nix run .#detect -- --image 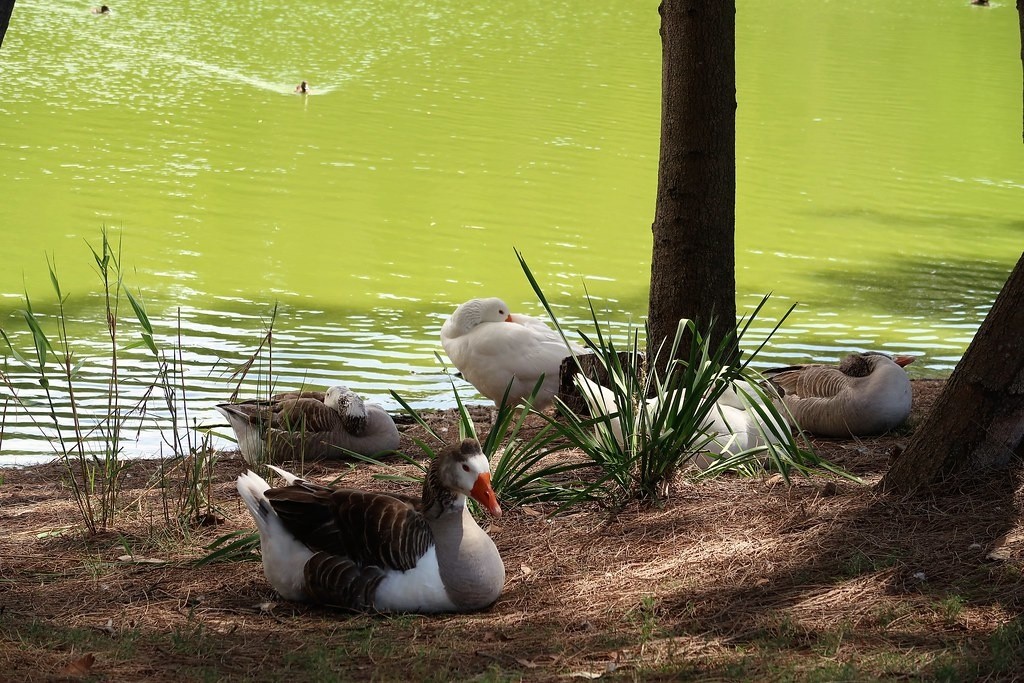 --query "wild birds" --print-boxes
[294,81,309,95]
[235,437,506,615]
[971,0,990,6]
[214,385,400,466]
[757,351,916,438]
[91,6,111,14]
[440,297,610,410]
[571,364,792,474]
